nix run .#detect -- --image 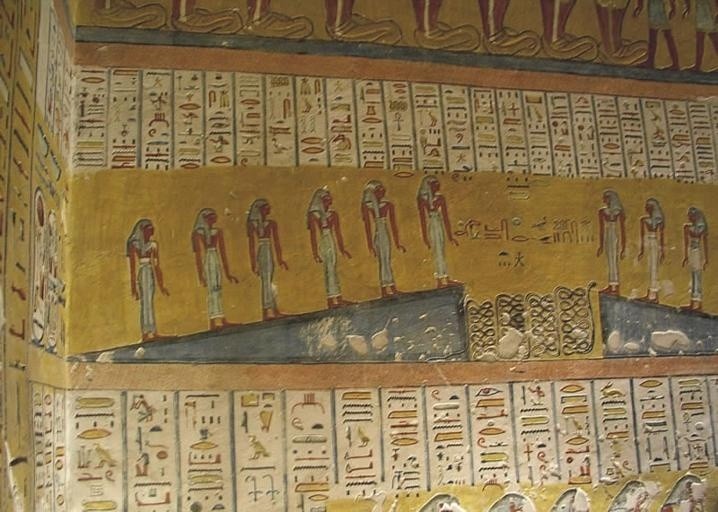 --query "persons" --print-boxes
[633,0,679,71]
[127,218,170,341]
[361,180,406,297]
[417,175,461,288]
[191,209,239,330]
[247,198,288,321]
[307,188,353,309]
[682,0,718,73]
[597,190,625,297]
[637,197,665,305]
[680,206,708,310]
